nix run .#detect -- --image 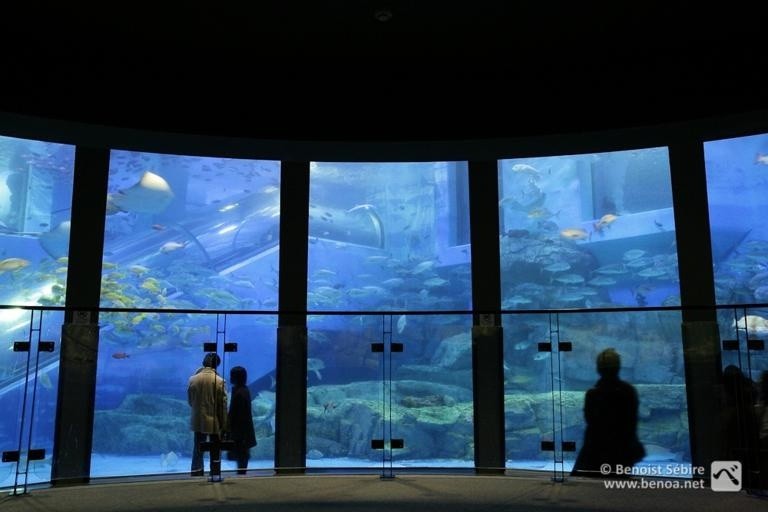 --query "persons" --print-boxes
[220,365,257,475]
[568,347,647,479]
[187,353,230,476]
